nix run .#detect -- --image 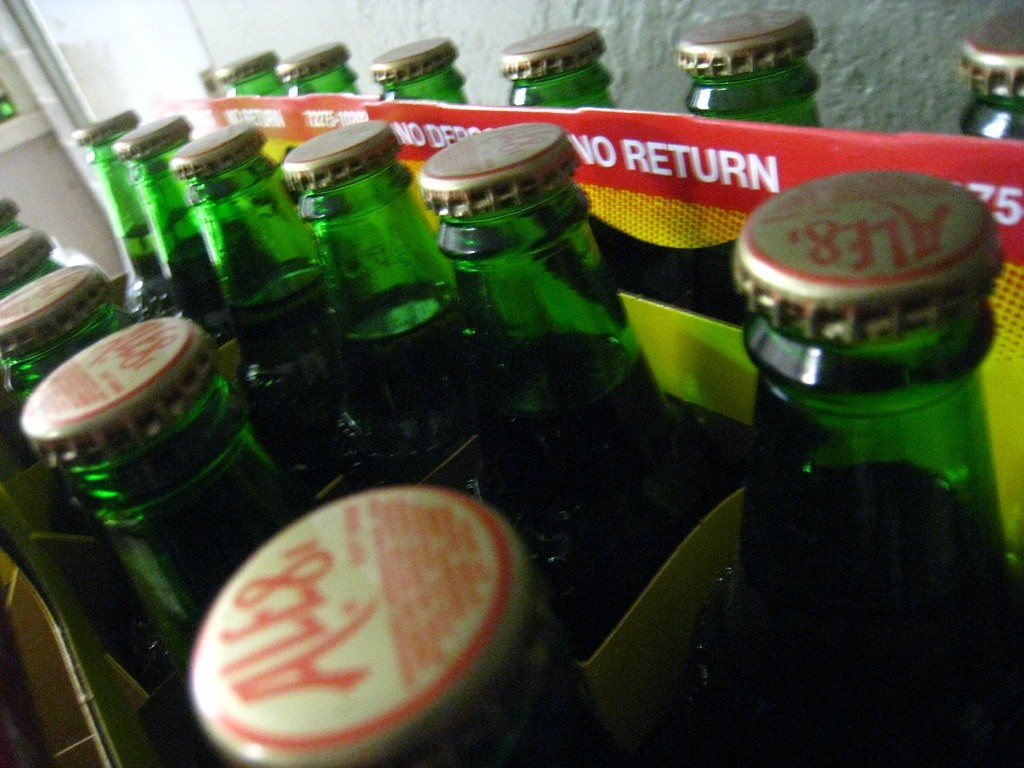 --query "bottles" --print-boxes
[0,10,1024,768]
